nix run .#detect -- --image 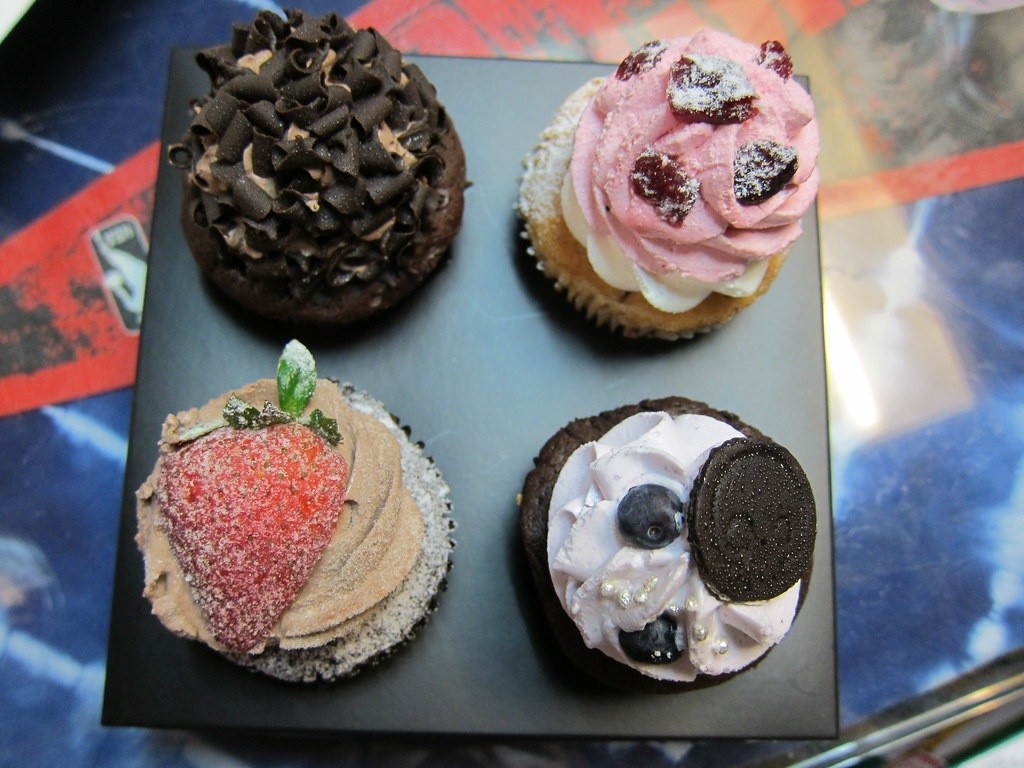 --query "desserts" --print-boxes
[179,7,468,326]
[133,339,455,683]
[519,397,818,692]
[517,26,819,343]
